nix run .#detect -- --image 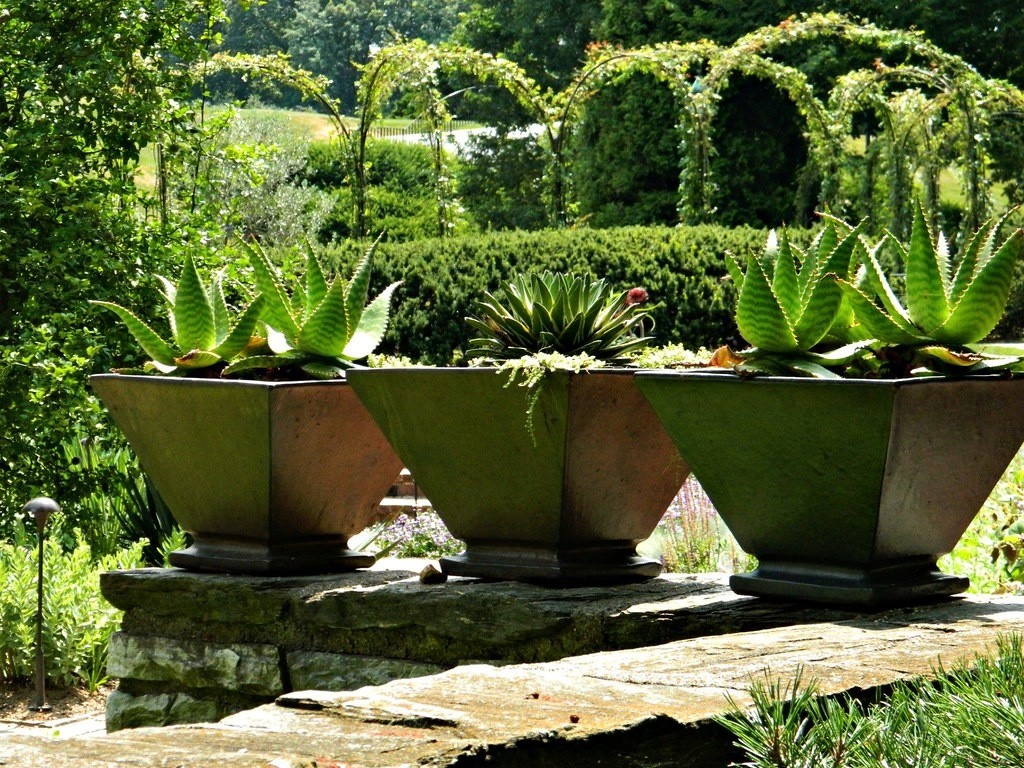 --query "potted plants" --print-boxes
[344,270,693,588]
[87,231,406,577]
[633,196,1023,606]
